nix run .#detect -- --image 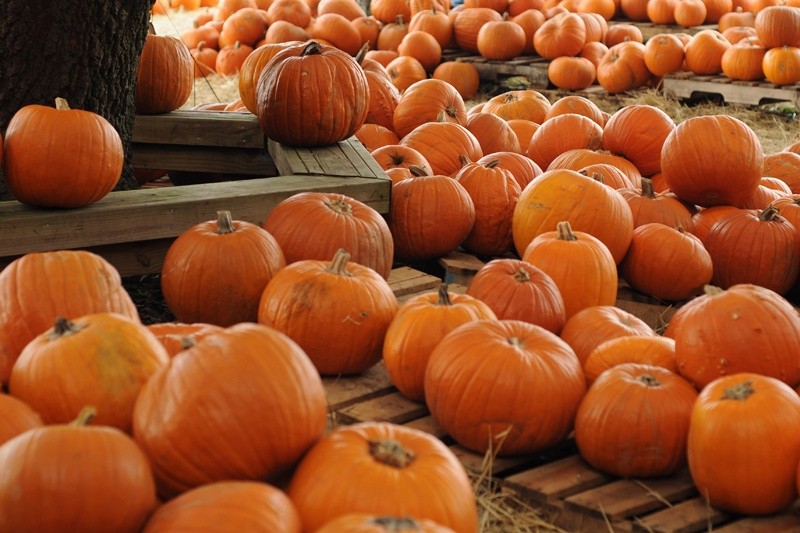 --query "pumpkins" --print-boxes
[0,0,800,533]
